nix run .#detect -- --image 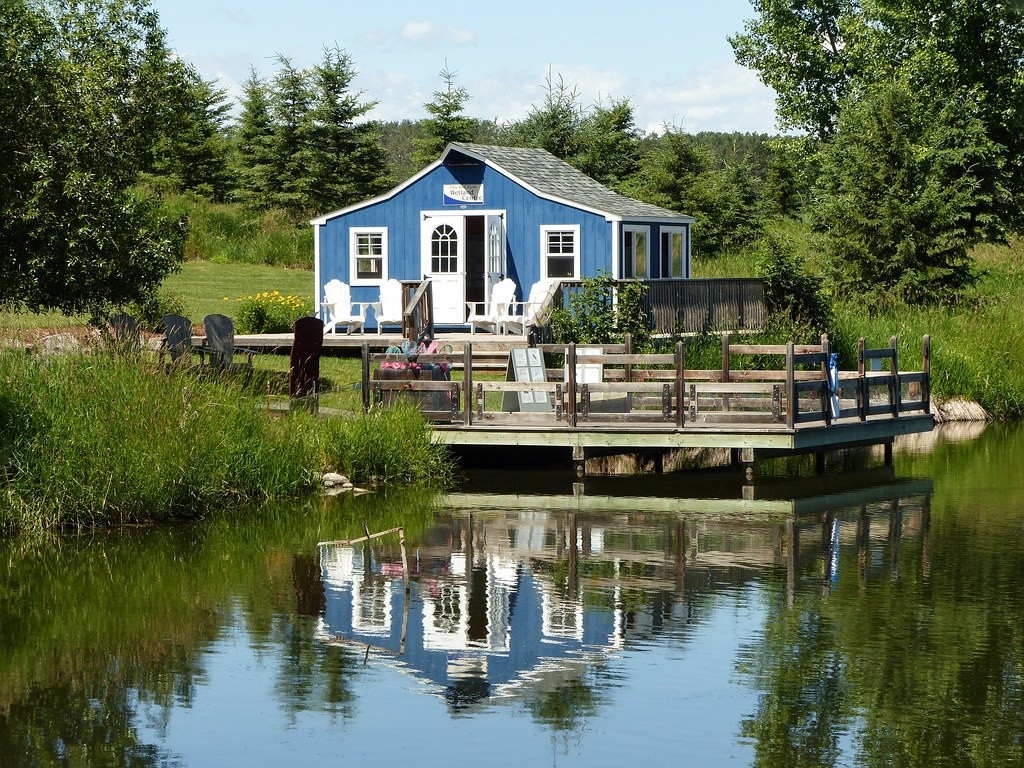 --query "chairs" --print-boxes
[320,279,371,335]
[159,315,218,365]
[371,278,401,334]
[497,278,562,336]
[197,314,260,388]
[465,278,517,335]
